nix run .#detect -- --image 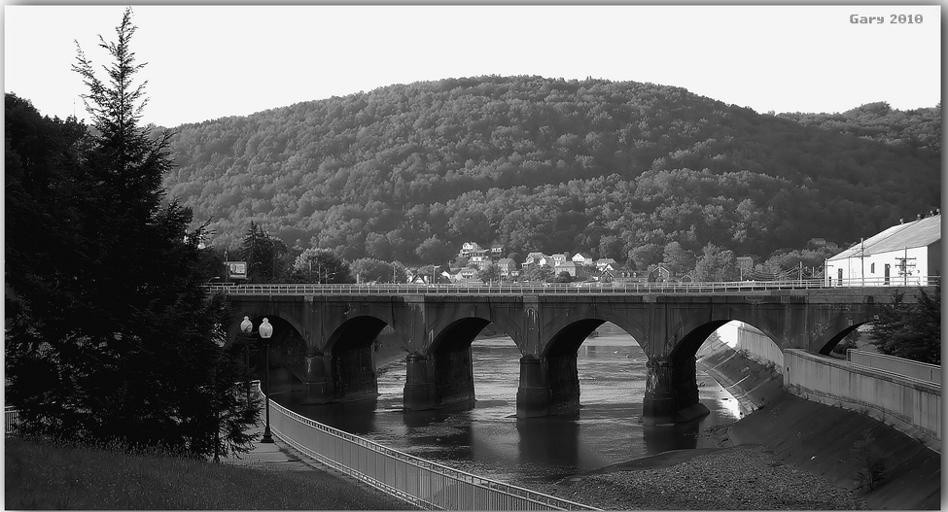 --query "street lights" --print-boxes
[206,276,221,287]
[256,318,276,444]
[325,271,335,283]
[239,315,253,403]
